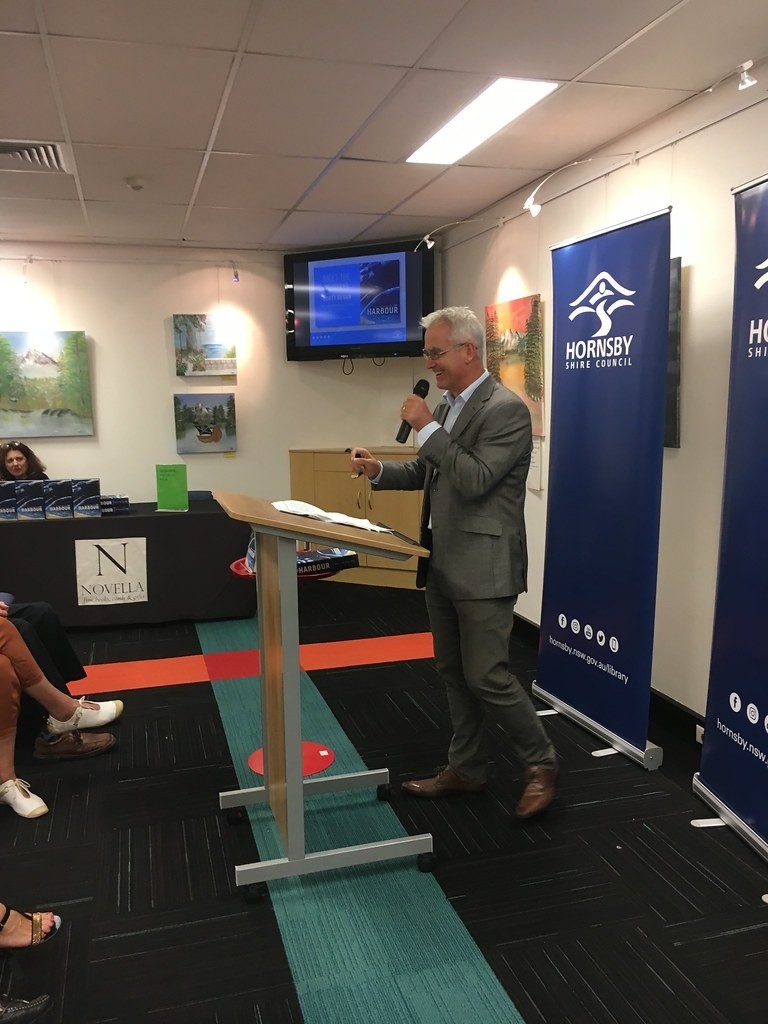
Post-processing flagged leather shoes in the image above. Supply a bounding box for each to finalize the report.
[515,763,561,818]
[400,765,487,797]
[32,726,116,758]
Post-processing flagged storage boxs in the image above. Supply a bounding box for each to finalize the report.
[296,547,358,575]
[246,530,257,574]
[0,478,130,520]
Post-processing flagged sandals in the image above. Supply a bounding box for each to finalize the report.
[0,901,62,947]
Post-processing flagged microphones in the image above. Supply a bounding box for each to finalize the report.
[396,379,430,444]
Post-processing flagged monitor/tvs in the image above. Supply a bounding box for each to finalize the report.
[284,237,436,361]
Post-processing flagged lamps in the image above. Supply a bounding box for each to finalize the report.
[523,150,637,217]
[230,259,239,282]
[710,55,768,91]
[414,214,506,252]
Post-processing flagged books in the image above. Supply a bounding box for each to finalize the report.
[245,529,257,573]
[297,548,360,574]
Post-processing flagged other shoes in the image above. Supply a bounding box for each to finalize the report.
[47,695,126,733]
[0,991,53,1024]
[0,774,49,819]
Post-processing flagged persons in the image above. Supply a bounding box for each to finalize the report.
[0,598,123,1024]
[350,307,562,820]
[0,441,49,481]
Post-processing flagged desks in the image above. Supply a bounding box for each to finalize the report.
[0,500,257,629]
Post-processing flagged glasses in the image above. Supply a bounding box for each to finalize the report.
[0,441,21,451]
[423,341,479,361]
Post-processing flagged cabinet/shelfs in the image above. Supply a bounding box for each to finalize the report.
[289,446,426,591]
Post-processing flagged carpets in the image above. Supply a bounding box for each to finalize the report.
[65,632,434,696]
[195,612,526,1024]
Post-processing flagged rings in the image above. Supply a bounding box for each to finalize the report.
[403,407,406,411]
